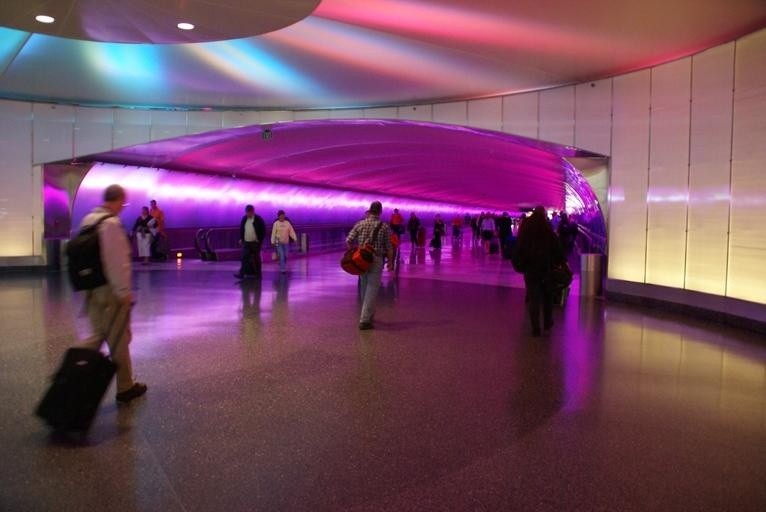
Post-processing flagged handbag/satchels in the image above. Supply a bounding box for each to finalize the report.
[341,243,376,275]
[66,224,107,292]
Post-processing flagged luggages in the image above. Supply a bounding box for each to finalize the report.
[34,299,136,441]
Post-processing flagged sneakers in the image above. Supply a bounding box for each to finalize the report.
[116,382,147,402]
[358,323,374,330]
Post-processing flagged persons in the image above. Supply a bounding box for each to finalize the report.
[346,200,393,331]
[273,210,295,243]
[232,204,266,279]
[511,206,561,338]
[129,206,158,265]
[342,208,579,284]
[75,184,148,402]
[149,200,164,260]
[270,211,298,275]
